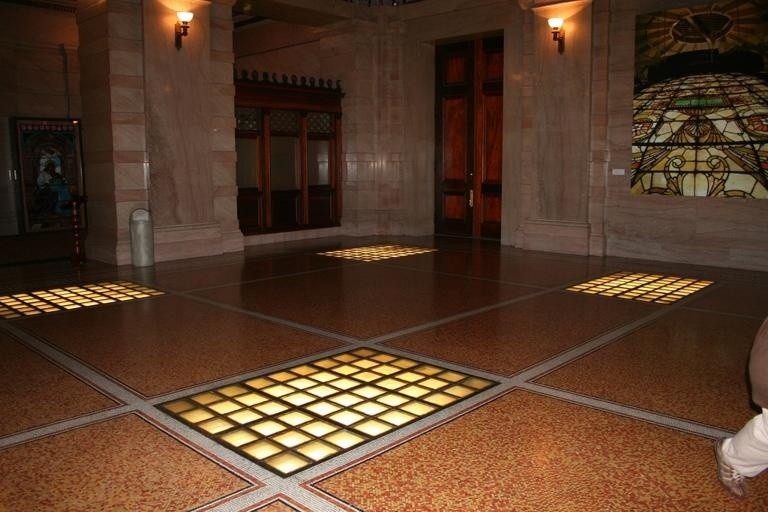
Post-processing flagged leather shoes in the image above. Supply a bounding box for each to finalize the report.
[714,437,746,498]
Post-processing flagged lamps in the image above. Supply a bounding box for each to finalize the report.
[548,17,565,53]
[175,10,194,50]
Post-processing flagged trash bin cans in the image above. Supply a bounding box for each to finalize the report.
[130,208,154,267]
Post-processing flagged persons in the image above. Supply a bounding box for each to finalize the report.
[31,158,68,229]
[712,317,768,499]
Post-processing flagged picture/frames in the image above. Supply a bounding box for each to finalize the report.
[9,113,88,232]
